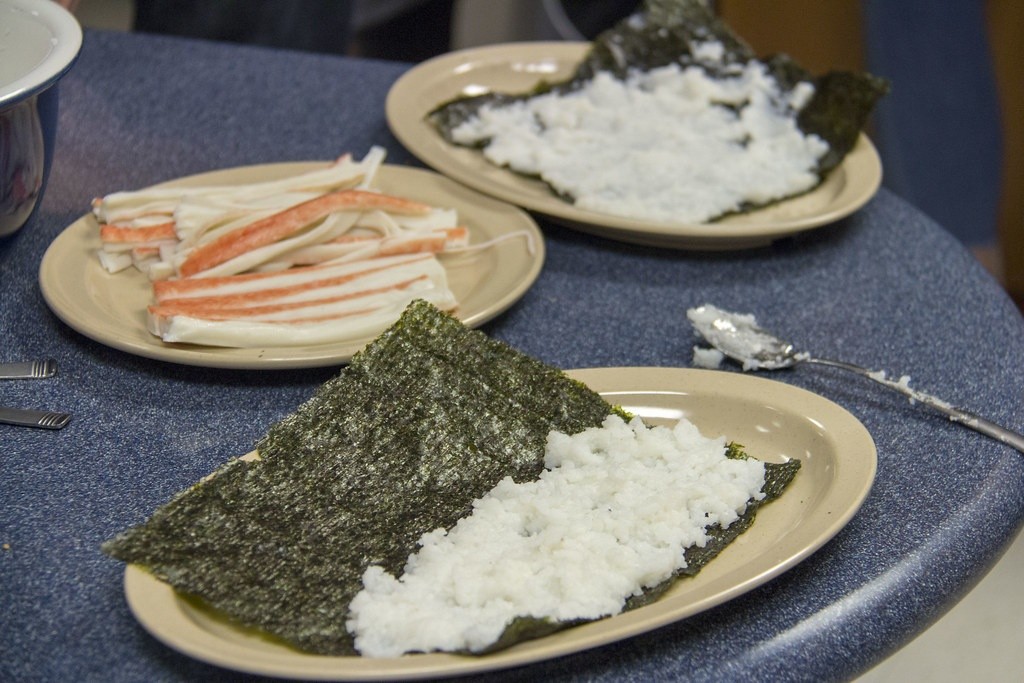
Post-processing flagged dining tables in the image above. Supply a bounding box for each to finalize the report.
[0,20,1023,683]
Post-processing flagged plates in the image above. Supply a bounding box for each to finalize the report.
[386,40,880,257]
[42,157,543,371]
[122,365,877,679]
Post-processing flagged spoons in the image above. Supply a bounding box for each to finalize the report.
[689,303,1022,464]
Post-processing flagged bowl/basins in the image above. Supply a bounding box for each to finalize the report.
[2,6,81,239]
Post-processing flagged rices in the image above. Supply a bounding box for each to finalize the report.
[345,413,767,657]
[450,40,831,224]
